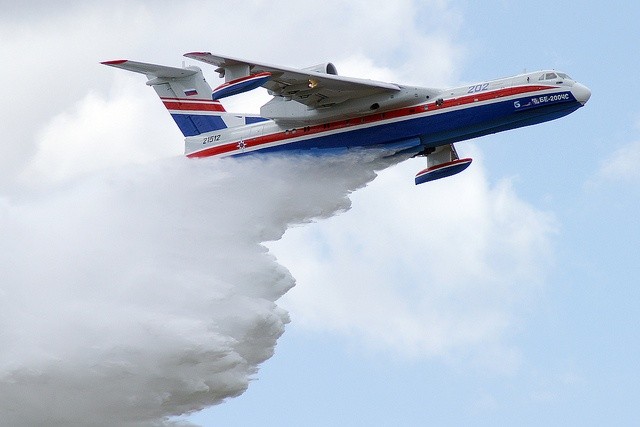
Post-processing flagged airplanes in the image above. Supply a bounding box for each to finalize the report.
[101,53,591,186]
